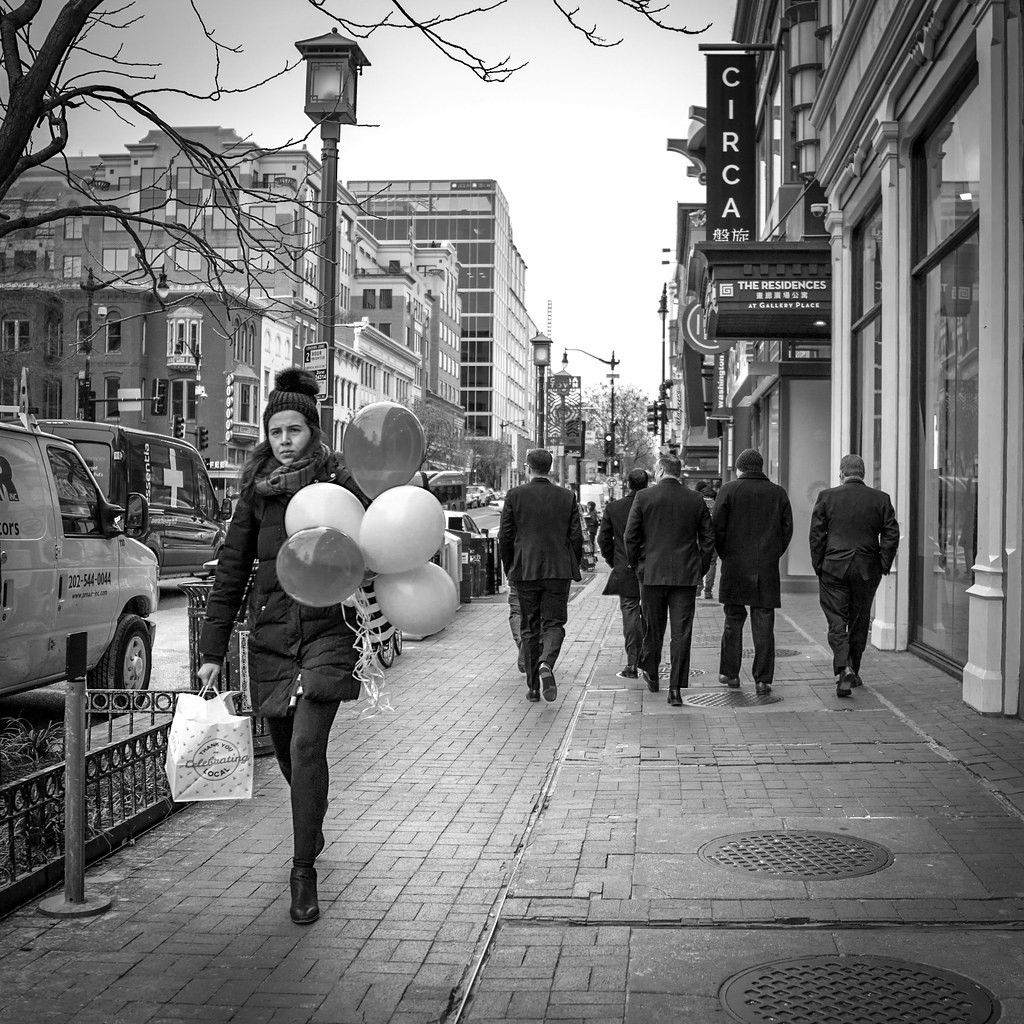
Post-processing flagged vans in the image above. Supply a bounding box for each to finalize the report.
[0,366,161,720]
[1,420,234,593]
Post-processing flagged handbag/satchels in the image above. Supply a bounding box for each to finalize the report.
[165,681,256,802]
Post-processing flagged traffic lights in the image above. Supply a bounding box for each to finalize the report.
[155,378,166,412]
[604,433,615,457]
[173,414,185,439]
[611,455,619,473]
[596,460,606,474]
[195,426,208,451]
[647,405,657,436]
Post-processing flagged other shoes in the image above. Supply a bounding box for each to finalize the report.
[517,643,526,672]
[539,663,558,703]
[526,688,539,704]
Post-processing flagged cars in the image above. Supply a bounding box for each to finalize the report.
[443,510,485,563]
[407,469,492,514]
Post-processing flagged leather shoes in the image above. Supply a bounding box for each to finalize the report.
[313,802,331,857]
[756,681,771,694]
[718,673,740,688]
[291,855,319,922]
[666,689,683,706]
[622,664,638,677]
[835,668,861,697]
[644,668,659,692]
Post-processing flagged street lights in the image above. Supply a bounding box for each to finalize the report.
[79,264,171,422]
[528,332,554,449]
[295,25,372,454]
[562,346,620,501]
[657,282,670,455]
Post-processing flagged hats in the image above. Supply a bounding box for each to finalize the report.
[734,449,763,474]
[263,368,323,428]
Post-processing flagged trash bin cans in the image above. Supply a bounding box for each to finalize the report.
[472,537,495,596]
[178,580,273,758]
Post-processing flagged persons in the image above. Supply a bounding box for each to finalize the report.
[586,501,599,543]
[809,455,900,697]
[713,449,794,695]
[624,455,713,705]
[695,481,717,599]
[501,449,584,702]
[951,477,974,550]
[597,468,649,680]
[198,369,375,925]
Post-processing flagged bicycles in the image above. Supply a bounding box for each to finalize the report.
[371,629,403,668]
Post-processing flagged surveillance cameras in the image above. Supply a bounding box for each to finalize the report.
[811,206,824,218]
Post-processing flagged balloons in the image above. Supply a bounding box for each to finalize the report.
[285,483,366,568]
[277,526,365,608]
[342,402,425,498]
[374,562,458,636]
[360,485,445,574]
